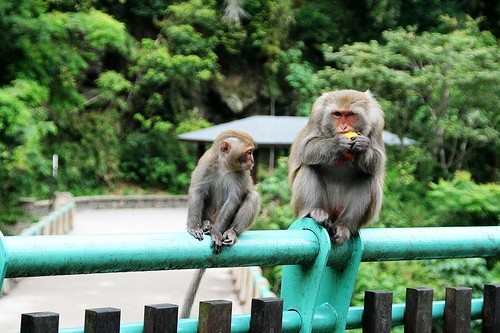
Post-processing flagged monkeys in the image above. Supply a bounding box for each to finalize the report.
[287,88,387,245]
[175,130,262,320]
